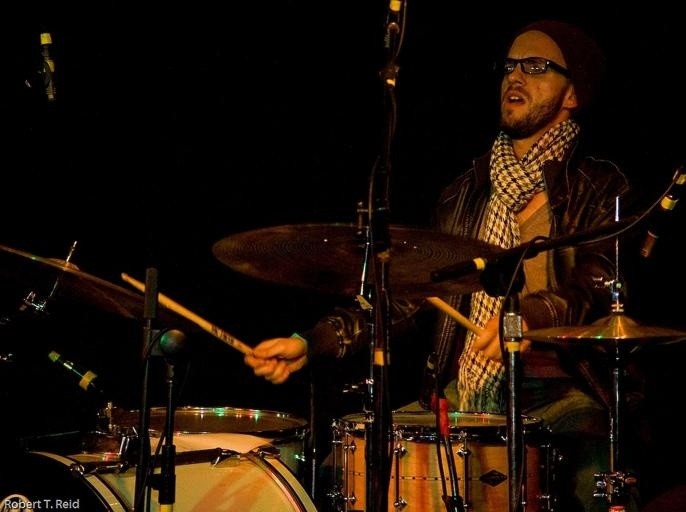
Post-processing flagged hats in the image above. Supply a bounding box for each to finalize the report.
[515,21,609,106]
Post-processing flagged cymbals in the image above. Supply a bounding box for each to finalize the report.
[212,223,508,300]
[0,244,173,320]
[520,317,685,349]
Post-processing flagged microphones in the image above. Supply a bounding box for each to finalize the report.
[158,365,175,504]
[40,32,57,101]
[378,0,402,77]
[640,169,685,258]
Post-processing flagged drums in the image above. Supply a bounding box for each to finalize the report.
[28,447,318,512]
[112,405,309,474]
[342,411,544,512]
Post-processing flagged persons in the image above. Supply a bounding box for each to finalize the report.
[244,20,635,512]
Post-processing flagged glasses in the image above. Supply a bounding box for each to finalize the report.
[498,57,569,76]
